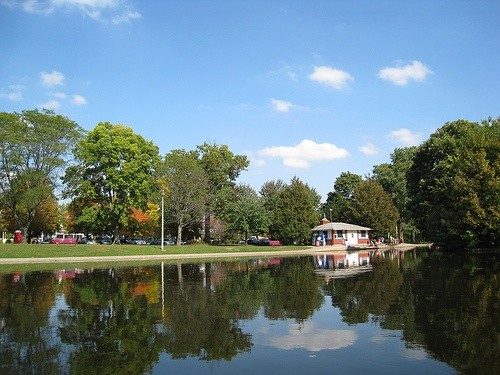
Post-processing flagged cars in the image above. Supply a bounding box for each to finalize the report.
[238,236,271,245]
[33,233,190,246]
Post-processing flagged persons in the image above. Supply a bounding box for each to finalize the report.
[368,250,405,261]
[367,235,403,247]
